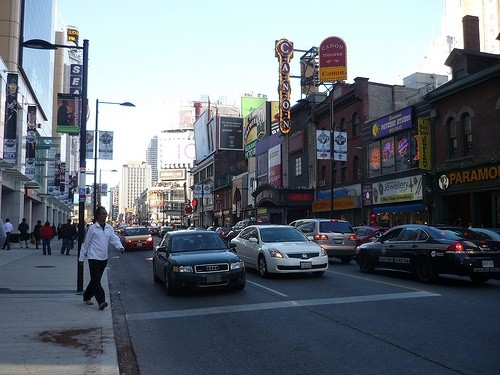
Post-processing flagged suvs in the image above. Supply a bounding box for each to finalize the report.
[288,218,357,265]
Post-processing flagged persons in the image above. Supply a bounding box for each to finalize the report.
[34,220,42,249]
[57,218,90,250]
[58,100,74,125]
[62,219,76,256]
[27,111,36,131]
[0,216,14,251]
[51,223,56,238]
[79,206,124,310]
[18,218,29,248]
[41,221,54,255]
[9,79,17,95]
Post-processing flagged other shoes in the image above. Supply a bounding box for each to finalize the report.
[99,302,108,310]
[83,297,93,305]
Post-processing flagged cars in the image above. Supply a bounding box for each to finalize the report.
[188,220,271,245]
[152,230,246,296]
[352,224,500,284]
[148,226,174,238]
[230,224,329,279]
[352,226,390,248]
[468,228,500,241]
[120,227,153,252]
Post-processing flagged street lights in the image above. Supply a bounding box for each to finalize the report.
[94,99,137,222]
[21,38,90,294]
[296,99,335,218]
[99,169,118,208]
